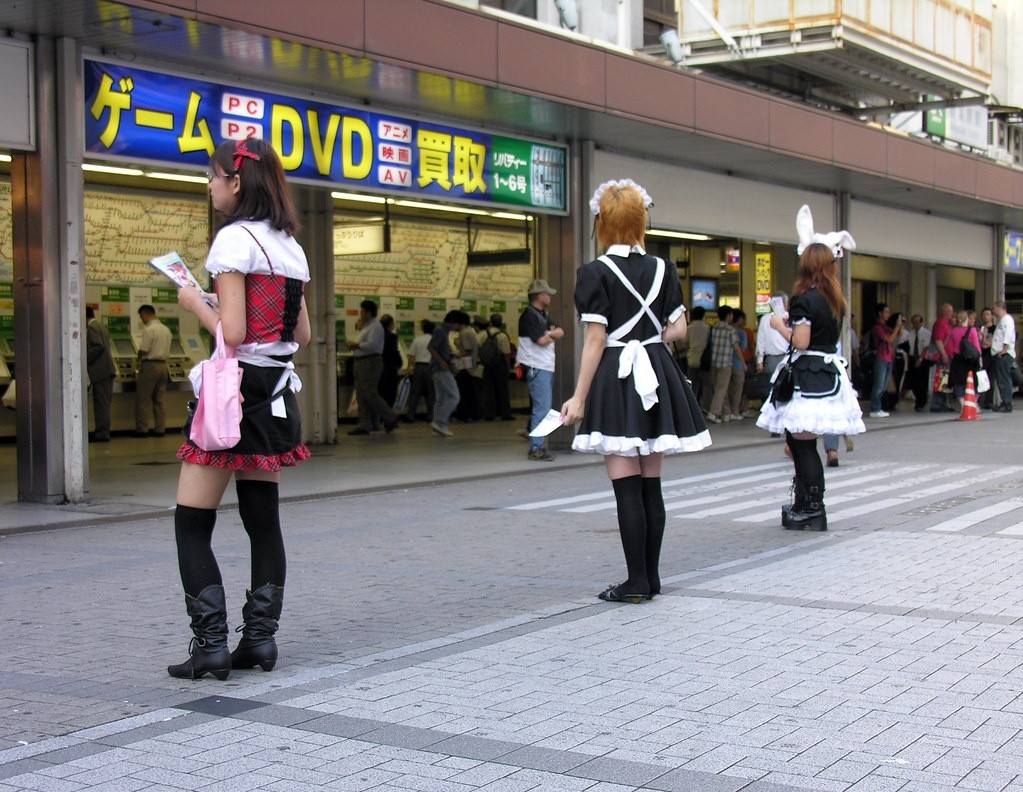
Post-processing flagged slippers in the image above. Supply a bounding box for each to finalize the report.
[598,582,656,603]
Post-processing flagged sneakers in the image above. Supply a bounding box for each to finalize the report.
[528,447,556,461]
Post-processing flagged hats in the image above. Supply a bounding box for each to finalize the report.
[527,279,557,295]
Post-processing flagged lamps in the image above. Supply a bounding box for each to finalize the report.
[555,0,579,31]
[660,29,686,69]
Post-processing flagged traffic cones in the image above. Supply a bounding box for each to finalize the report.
[957,370,982,421]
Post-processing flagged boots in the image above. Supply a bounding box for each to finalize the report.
[168,584,232,681]
[781,474,826,530]
[230,582,285,671]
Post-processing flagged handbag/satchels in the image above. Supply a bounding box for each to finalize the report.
[958,326,980,366]
[700,326,714,372]
[933,366,955,393]
[393,375,411,412]
[189,321,245,450]
[921,344,943,361]
[771,334,795,403]
[976,369,990,392]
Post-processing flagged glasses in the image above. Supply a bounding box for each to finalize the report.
[205,171,236,185]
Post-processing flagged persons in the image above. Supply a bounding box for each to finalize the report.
[756,204,866,530]
[133,304,173,438]
[346,300,516,437]
[167,137,311,680]
[516,278,564,463]
[86,305,117,443]
[681,305,792,426]
[851,301,1016,418]
[561,178,713,602]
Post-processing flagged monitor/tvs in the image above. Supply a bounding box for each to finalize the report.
[114,339,136,355]
[7,338,15,352]
[336,340,350,352]
[168,338,185,355]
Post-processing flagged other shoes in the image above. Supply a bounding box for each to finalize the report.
[826,450,839,467]
[429,422,454,437]
[131,430,165,438]
[347,415,416,435]
[693,398,758,422]
[785,445,794,460]
[866,385,1023,418]
[88,434,111,442]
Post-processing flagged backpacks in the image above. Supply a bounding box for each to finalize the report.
[479,330,504,366]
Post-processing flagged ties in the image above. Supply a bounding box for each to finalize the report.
[913,329,919,358]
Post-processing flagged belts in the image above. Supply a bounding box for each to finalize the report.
[142,358,166,364]
